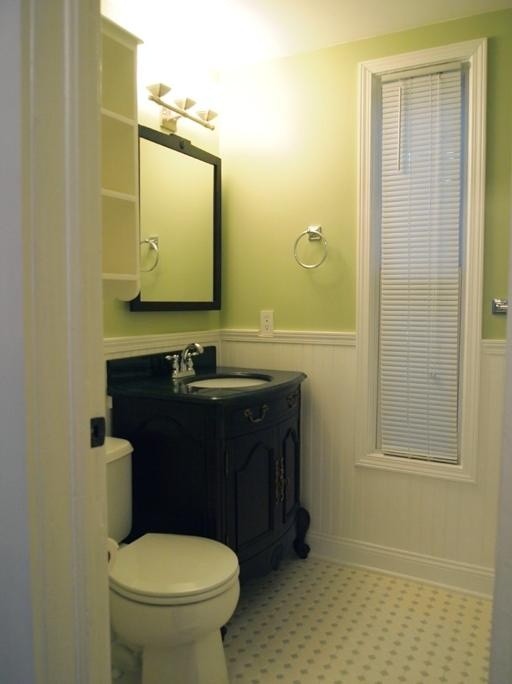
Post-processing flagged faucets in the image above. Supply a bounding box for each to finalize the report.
[180,342,205,374]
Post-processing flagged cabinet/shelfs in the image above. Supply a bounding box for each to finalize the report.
[110,412,300,566]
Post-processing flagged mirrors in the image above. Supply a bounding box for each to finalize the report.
[130,124,222,309]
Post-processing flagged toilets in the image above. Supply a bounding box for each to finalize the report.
[103,436,241,684]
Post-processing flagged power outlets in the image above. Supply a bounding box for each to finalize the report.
[262,311,273,336]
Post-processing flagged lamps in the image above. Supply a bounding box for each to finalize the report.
[147,69,217,133]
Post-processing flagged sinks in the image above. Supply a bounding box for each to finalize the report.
[183,373,274,388]
